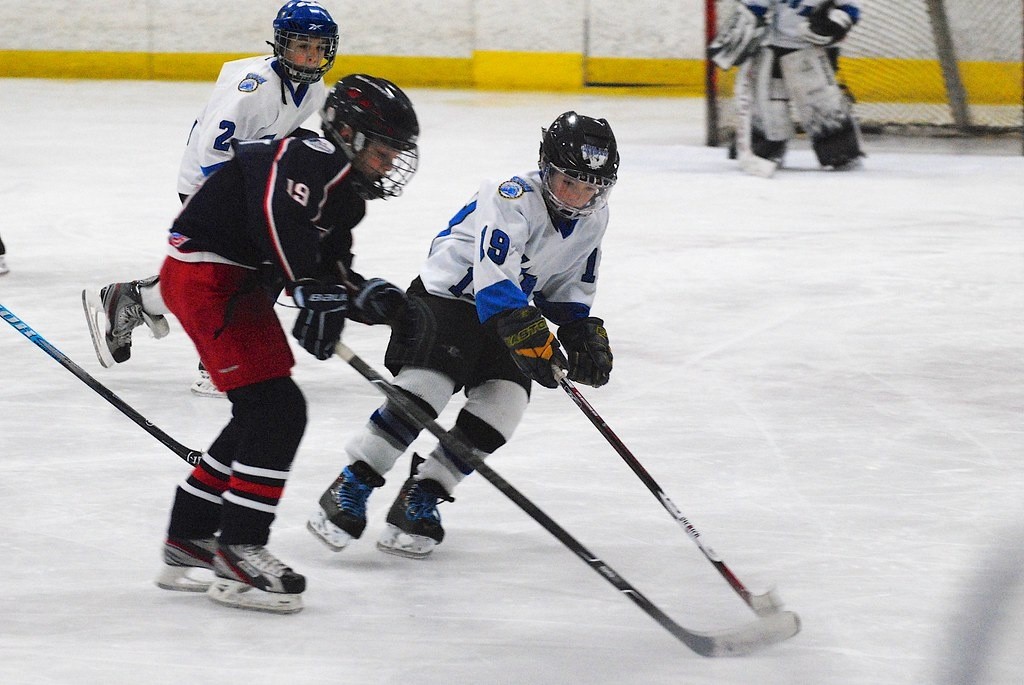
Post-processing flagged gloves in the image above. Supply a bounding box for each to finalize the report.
[496,306,570,389]
[557,317,613,388]
[293,283,348,361]
[354,277,413,343]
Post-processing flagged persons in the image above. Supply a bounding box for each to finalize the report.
[705,0,867,176]
[158,74,419,614]
[308,106,620,559]
[81,0,339,397]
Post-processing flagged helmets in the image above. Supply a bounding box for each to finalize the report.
[537,112,620,222]
[272,1,339,84]
[321,73,419,200]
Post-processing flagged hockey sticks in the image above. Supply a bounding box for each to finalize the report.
[0,303,207,464]
[552,364,828,617]
[335,343,802,659]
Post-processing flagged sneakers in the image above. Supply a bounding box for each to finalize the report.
[377,452,455,558]
[153,535,221,592]
[206,542,306,615]
[81,274,169,367]
[190,358,228,398]
[308,459,386,547]
